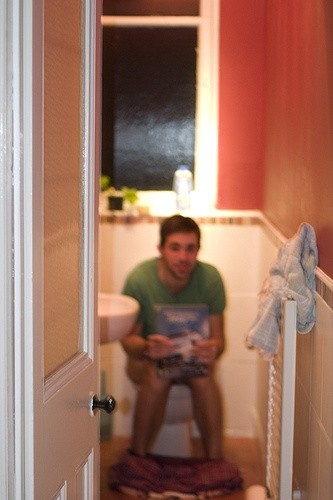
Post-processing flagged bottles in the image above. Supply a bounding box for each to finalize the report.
[171,164,193,211]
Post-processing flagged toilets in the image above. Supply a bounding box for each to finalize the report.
[147,382,195,458]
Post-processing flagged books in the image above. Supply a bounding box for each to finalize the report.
[151,302,210,381]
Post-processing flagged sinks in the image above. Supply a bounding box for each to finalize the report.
[99,294,137,315]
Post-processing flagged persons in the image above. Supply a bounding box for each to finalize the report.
[118,214,225,464]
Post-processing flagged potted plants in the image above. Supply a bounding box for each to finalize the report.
[100,175,138,210]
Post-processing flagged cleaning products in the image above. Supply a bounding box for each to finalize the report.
[172,159,193,210]
